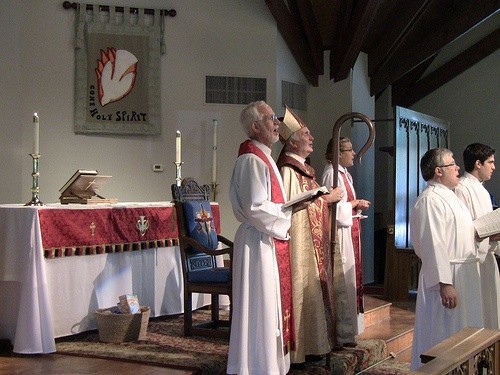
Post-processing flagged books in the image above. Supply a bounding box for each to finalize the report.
[283,186,330,208]
[59,168,99,194]
[474,208,500,238]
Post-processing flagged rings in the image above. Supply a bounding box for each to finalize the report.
[446,301,449,304]
[340,194,342,198]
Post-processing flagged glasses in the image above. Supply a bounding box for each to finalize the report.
[263,116,277,120]
[438,163,455,167]
[340,149,353,152]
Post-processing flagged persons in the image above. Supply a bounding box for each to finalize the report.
[410,148,485,371]
[321,137,371,352]
[276,104,344,371]
[227,101,311,375]
[452,142,500,330]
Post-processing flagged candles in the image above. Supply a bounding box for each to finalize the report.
[175,130,182,162]
[33,113,39,154]
[212,119,217,182]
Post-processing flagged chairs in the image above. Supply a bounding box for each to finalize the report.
[172,177,234,337]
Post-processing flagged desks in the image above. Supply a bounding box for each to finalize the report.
[0,200,230,355]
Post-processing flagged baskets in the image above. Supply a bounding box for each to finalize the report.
[95,306,151,342]
